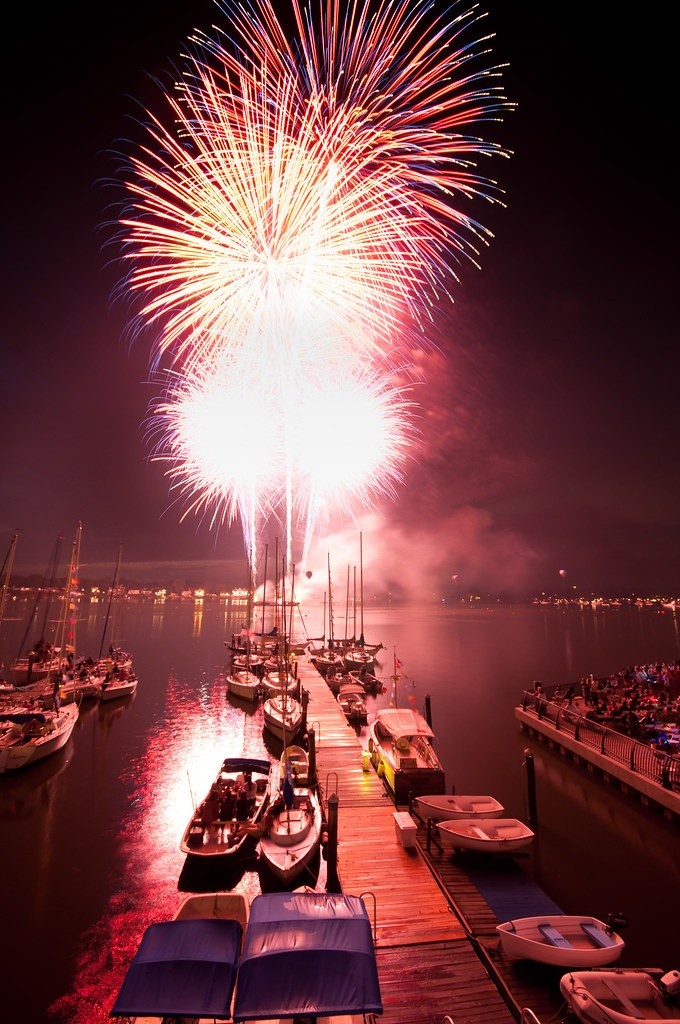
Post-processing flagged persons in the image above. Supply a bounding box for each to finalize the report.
[359,665,366,681]
[231,633,247,648]
[78,651,135,680]
[319,646,326,658]
[196,776,257,832]
[531,660,680,751]
[329,664,336,681]
[23,696,35,708]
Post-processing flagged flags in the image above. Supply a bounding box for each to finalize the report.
[60,565,79,684]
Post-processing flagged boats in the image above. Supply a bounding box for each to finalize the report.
[558,967,680,1024]
[415,793,507,825]
[176,756,274,862]
[227,886,387,1024]
[109,892,250,1023]
[493,913,627,971]
[431,817,534,851]
[366,707,446,799]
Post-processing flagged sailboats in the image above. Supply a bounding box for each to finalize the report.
[0,520,139,772]
[224,534,389,886]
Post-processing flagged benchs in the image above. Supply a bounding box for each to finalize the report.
[539,924,573,948]
[449,800,463,811]
[580,924,613,949]
[603,978,645,1018]
[195,818,252,825]
[472,825,490,840]
[342,702,361,705]
